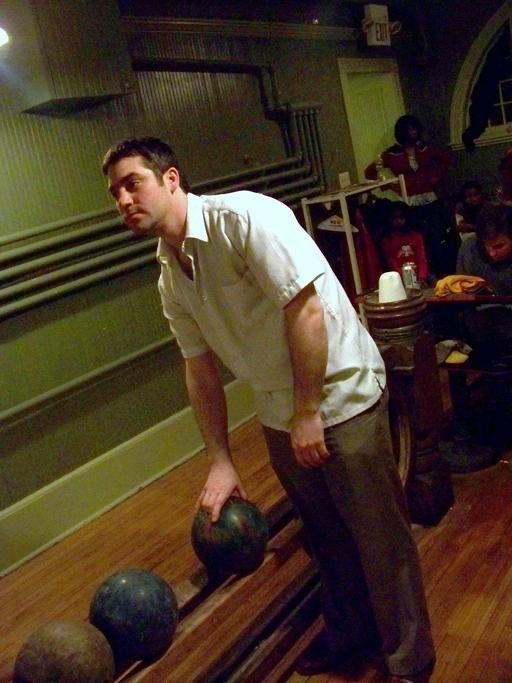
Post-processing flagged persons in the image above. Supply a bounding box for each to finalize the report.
[363,116,512,371]
[102,136,436,683]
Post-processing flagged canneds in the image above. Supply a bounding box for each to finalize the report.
[402,261,417,288]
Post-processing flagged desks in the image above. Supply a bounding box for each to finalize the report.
[418,279,511,475]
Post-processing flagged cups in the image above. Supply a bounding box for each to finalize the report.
[379,272,407,302]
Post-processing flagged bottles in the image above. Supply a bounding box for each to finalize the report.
[376,155,385,181]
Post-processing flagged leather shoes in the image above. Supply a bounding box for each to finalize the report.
[386,655,436,683]
[295,644,341,676]
